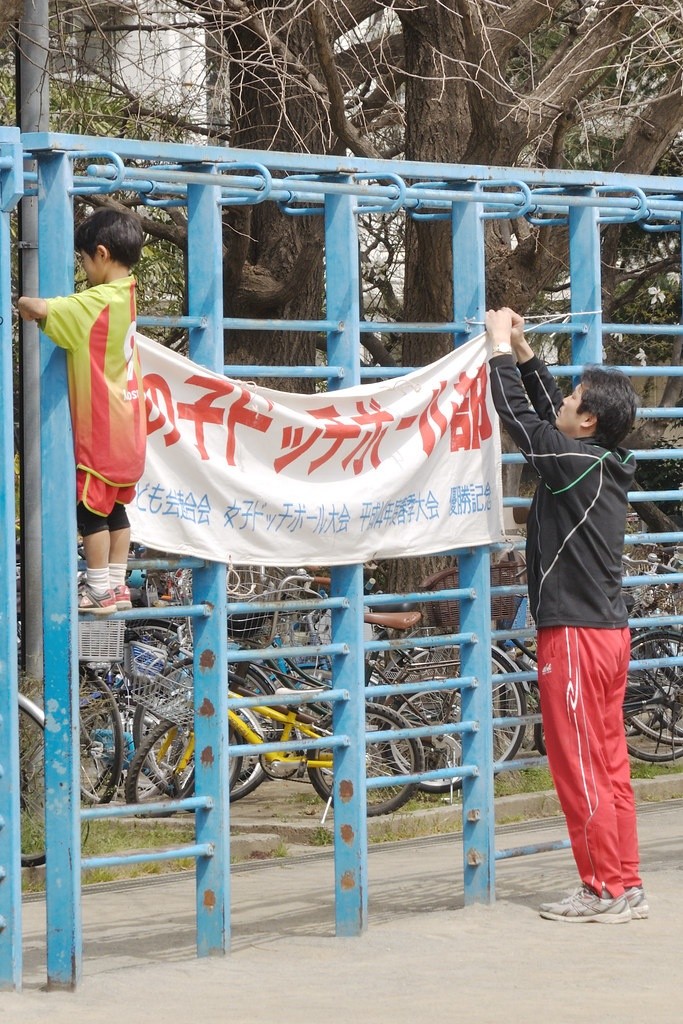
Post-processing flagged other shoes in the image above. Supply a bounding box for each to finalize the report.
[78,580,116,614]
[114,584,132,609]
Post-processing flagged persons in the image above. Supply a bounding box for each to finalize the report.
[15,206,149,615]
[480,306,651,926]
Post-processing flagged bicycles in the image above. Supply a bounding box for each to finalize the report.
[15,537,683,828]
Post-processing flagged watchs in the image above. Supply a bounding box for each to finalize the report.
[491,342,513,354]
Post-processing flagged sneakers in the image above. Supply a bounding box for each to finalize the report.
[626,886,649,919]
[538,890,631,924]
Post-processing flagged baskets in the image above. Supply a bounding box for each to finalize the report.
[68,554,654,725]
